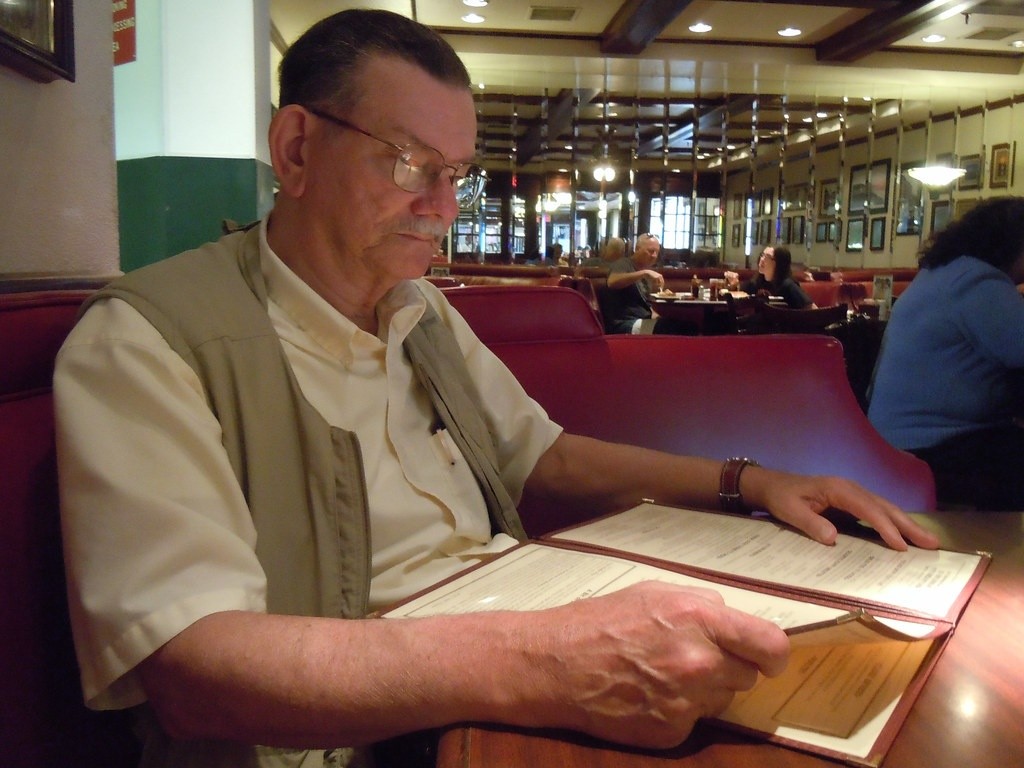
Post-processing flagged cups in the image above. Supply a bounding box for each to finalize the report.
[691,279,703,297]
[710,279,727,298]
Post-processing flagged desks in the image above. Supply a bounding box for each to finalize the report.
[437,505,1024,768]
[645,289,779,334]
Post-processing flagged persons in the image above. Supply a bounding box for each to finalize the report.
[52,8,938,768]
[545,237,634,267]
[581,238,625,268]
[868,196,1024,512]
[607,233,665,320]
[724,242,818,310]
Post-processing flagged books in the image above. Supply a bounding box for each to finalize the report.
[368,496,992,768]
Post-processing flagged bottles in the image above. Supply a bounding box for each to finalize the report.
[877,299,889,316]
[698,286,705,298]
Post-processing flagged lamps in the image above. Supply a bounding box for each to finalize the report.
[906,161,966,193]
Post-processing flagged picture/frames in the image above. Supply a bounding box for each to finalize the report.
[895,159,923,235]
[989,140,1015,187]
[869,216,884,249]
[929,201,953,236]
[0,0,80,86]
[935,149,955,189]
[698,202,719,244]
[729,179,840,247]
[959,152,985,191]
[845,217,864,252]
[849,159,890,214]
[956,198,984,218]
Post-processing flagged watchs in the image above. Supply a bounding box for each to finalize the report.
[719,456,761,515]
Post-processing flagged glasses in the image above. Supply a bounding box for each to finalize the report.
[759,251,775,261]
[646,232,660,239]
[302,103,492,210]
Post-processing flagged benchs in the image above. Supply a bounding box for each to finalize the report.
[0,265,1024,768]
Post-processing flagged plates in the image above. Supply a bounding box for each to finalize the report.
[650,292,680,298]
